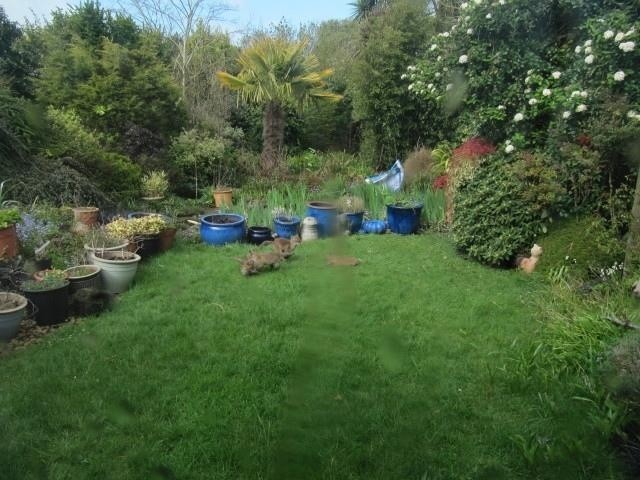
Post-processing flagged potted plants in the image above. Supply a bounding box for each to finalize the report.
[196,186,425,247]
[1,204,177,346]
